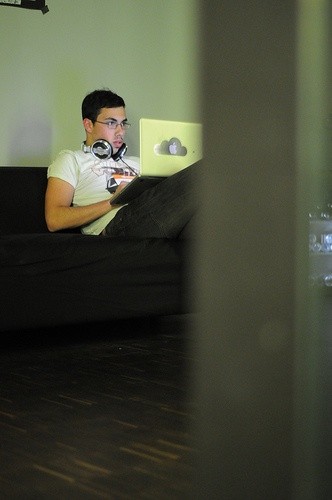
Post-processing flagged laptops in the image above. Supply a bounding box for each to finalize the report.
[109,119,202,205]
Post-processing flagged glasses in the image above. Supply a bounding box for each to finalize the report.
[94,121,130,129]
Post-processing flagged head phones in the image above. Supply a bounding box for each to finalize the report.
[80,141,128,161]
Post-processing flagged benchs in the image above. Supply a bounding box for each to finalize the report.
[0,167,194,328]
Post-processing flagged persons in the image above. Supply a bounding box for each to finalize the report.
[44,87,214,240]
